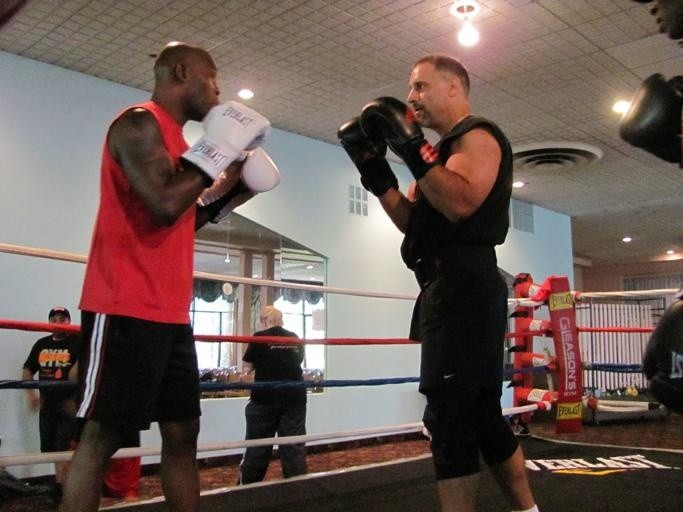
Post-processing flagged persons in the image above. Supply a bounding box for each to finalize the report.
[54,41,282,510]
[20,305,82,501]
[333,51,547,511]
[237,304,309,486]
[617,1,683,418]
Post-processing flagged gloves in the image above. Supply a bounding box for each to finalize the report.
[178,100,271,189]
[336,116,398,198]
[196,141,280,224]
[619,71,683,165]
[641,288,683,417]
[359,97,440,181]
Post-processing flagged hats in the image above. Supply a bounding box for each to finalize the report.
[48,306,69,318]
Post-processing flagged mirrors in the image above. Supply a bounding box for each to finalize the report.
[189,210,330,402]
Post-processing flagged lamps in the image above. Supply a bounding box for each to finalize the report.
[449,0,480,47]
